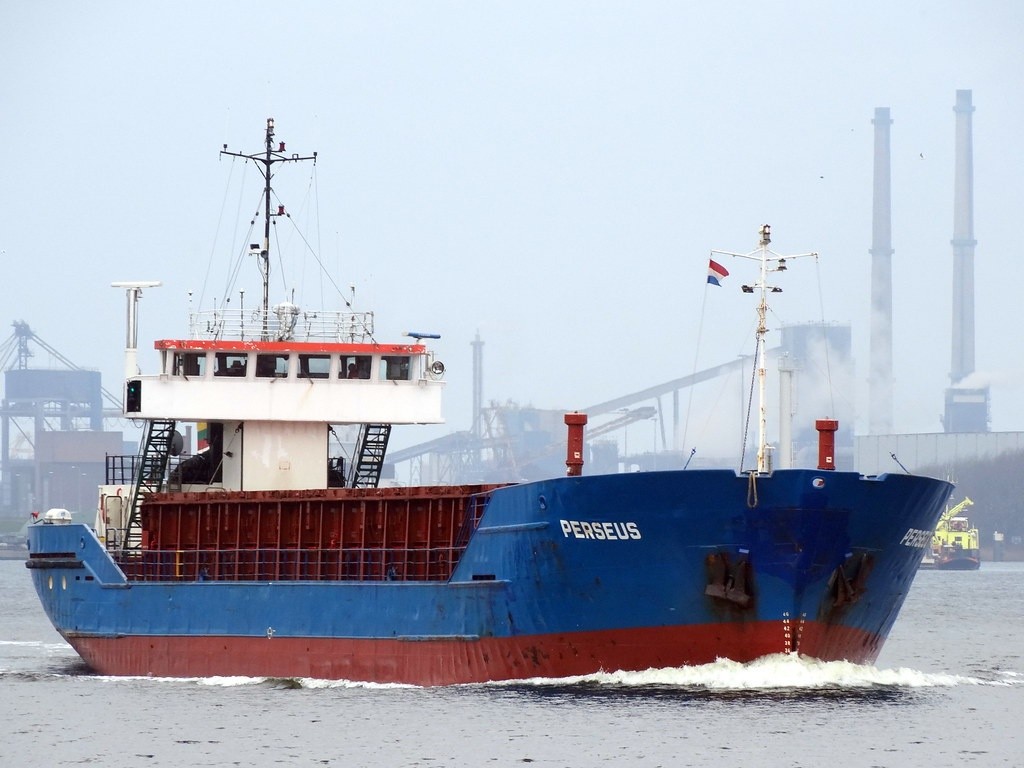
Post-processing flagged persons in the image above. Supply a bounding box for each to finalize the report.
[347,363,359,379]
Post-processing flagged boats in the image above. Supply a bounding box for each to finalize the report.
[927,471,981,570]
[21,115,957,687]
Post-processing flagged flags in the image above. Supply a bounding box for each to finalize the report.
[197,422,211,450]
[707,259,730,287]
